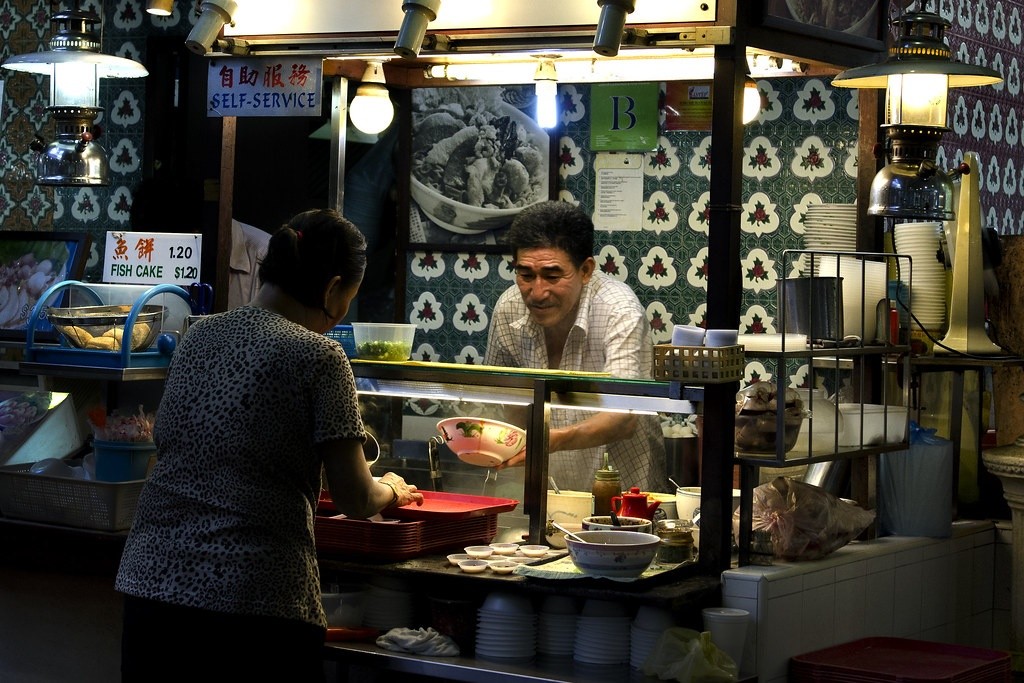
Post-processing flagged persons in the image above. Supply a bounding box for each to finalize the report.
[484,200,668,493]
[113,209,423,683]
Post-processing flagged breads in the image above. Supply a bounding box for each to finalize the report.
[62,322,152,351]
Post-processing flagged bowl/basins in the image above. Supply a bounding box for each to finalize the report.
[475,594,675,668]
[435,416,527,467]
[735,403,908,453]
[410,101,552,234]
[352,322,417,362]
[564,485,742,577]
[819,256,886,345]
[30,453,94,492]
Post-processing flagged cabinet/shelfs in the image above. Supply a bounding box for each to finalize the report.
[346,364,714,607]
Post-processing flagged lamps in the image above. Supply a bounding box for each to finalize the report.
[145,0,175,16]
[393,0,452,59]
[743,76,761,125]
[2,0,149,185]
[356,378,697,417]
[831,0,1004,220]
[593,0,654,57]
[186,0,249,56]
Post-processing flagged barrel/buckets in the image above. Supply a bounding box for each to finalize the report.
[90,439,157,482]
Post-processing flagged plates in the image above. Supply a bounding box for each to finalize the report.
[361,587,413,630]
[0,396,49,427]
[447,543,549,574]
[800,204,948,331]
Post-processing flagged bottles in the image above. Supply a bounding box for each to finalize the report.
[790,389,846,450]
[595,450,621,518]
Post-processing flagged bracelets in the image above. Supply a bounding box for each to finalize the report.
[380,482,399,506]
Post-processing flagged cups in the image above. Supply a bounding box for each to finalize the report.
[665,437,697,493]
[545,490,592,548]
[776,277,843,342]
[665,324,739,374]
[703,607,750,680]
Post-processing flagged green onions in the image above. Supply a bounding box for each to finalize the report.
[354,340,411,361]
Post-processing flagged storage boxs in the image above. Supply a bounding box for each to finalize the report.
[833,402,909,447]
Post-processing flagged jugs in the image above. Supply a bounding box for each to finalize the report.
[611,487,663,522]
